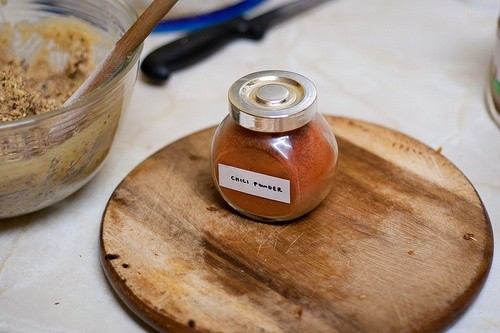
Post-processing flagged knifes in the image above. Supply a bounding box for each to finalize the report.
[138,0,340,86]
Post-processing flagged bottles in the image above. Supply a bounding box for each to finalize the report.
[209,68,339,224]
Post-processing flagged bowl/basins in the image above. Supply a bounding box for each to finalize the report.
[0,0,144,218]
